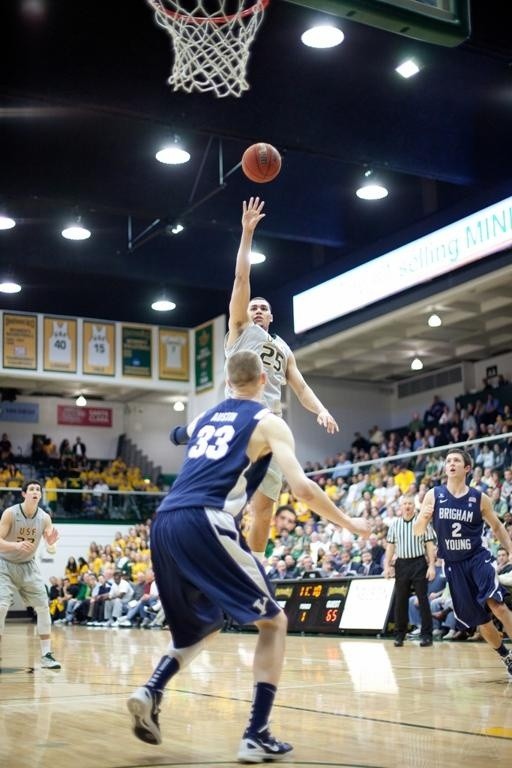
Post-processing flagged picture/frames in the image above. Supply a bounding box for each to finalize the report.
[158,327,190,382]
[83,319,116,377]
[194,320,213,394]
[122,322,152,376]
[43,316,78,374]
[2,312,38,369]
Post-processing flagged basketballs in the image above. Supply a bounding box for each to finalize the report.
[243,144,281,183]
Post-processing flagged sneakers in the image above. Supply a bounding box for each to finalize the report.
[125,685,162,745]
[499,649,511,682]
[41,652,61,670]
[53,617,171,629]
[237,728,294,764]
[394,638,404,647]
[407,627,483,641]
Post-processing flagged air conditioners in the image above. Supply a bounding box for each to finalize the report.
[390,361,476,429]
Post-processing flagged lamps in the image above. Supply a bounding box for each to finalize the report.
[410,349,424,371]
[0,200,16,230]
[301,12,345,49]
[427,305,442,327]
[61,207,92,241]
[155,132,191,165]
[0,262,22,294]
[75,393,87,407]
[249,233,266,265]
[151,288,176,312]
[356,161,388,200]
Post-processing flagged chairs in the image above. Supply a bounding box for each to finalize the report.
[0,454,167,520]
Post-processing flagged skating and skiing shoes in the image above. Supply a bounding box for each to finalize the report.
[419,637,433,646]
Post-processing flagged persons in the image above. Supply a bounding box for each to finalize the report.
[39,456,160,520]
[384,497,436,647]
[46,515,170,631]
[0,478,60,669]
[241,375,512,583]
[413,448,512,674]
[407,547,512,640]
[60,436,86,464]
[30,433,56,461]
[1,433,25,489]
[224,196,339,565]
[125,350,371,763]
